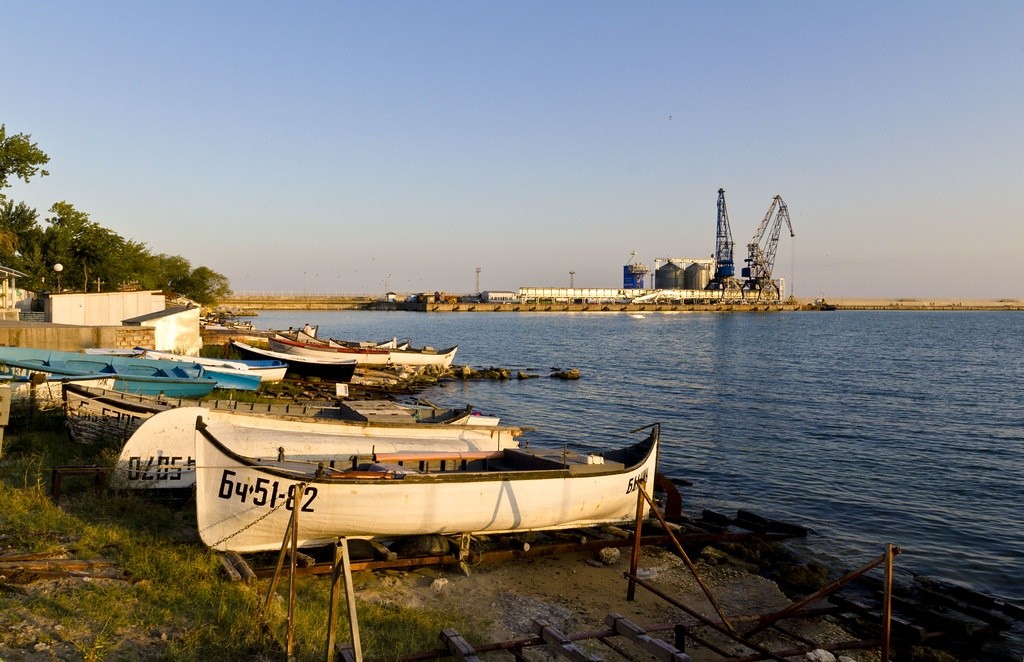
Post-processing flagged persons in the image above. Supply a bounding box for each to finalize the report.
[304,323,311,335]
[289,327,293,333]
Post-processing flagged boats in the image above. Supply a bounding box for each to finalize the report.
[61,376,502,449]
[228,337,360,384]
[274,331,459,367]
[267,335,391,370]
[276,325,320,339]
[108,405,538,494]
[192,415,662,554]
[1,369,119,412]
[0,346,263,391]
[0,352,219,400]
[79,344,289,386]
[199,312,257,332]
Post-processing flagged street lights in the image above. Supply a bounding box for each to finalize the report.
[475,267,482,296]
[569,271,576,289]
[54,263,64,294]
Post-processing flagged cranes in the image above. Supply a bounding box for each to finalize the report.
[703,186,740,305]
[737,194,796,302]
